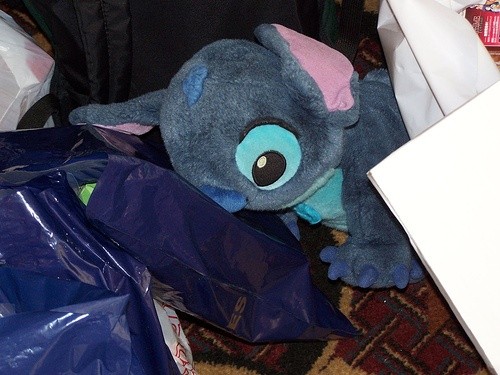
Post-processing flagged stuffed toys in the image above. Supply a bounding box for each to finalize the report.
[68,22,428,290]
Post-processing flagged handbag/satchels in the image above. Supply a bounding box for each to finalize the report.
[0,162,203,375]
[2,115,369,346]
[366,0,499,375]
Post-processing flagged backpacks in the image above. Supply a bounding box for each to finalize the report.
[20,2,361,155]
